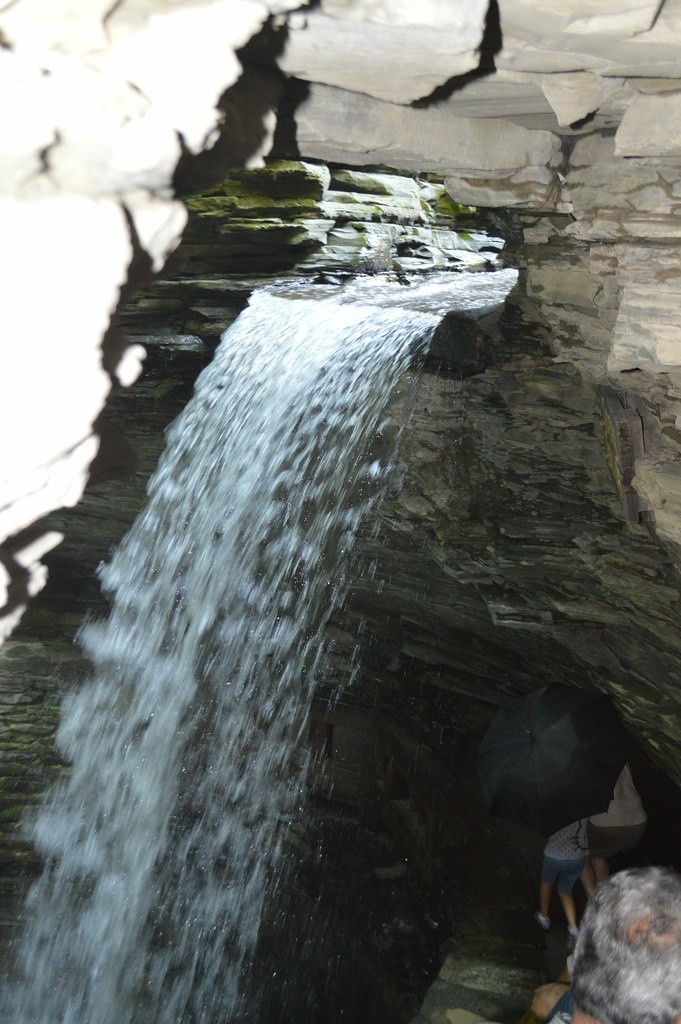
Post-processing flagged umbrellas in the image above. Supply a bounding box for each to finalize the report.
[468,682,632,838]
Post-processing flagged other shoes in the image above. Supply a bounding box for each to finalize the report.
[534,911,550,929]
[567,926,579,935]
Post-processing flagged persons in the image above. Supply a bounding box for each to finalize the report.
[536,760,648,955]
